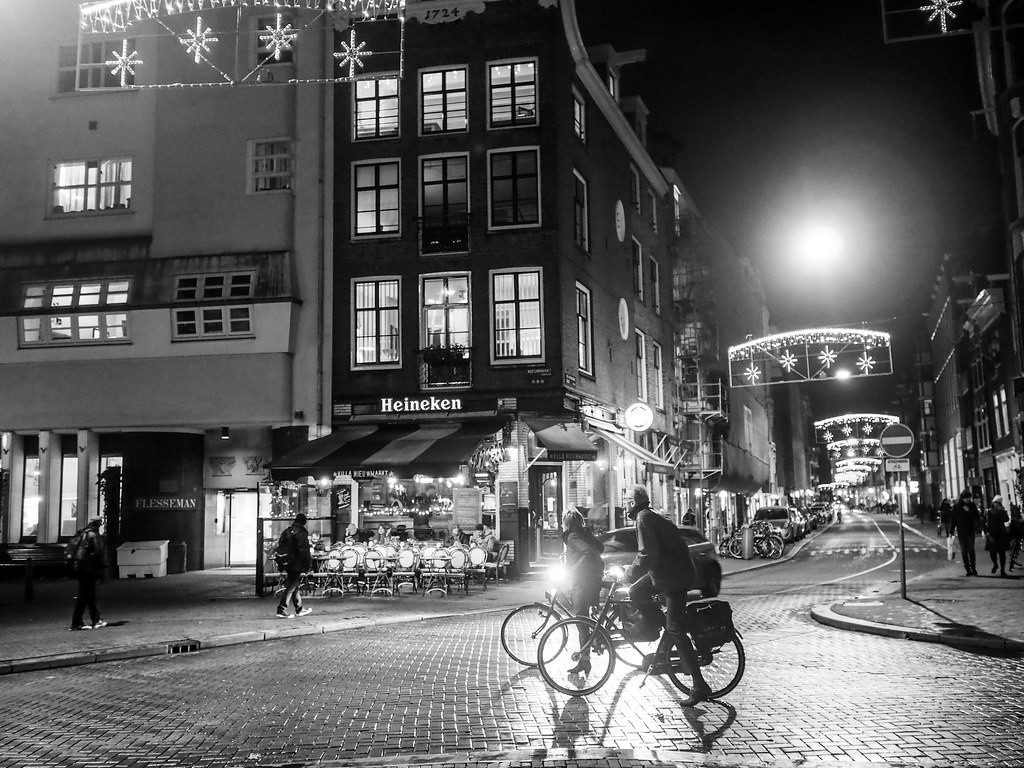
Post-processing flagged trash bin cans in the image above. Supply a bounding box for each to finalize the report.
[742,528,754,560]
[166,541,188,573]
[116,540,170,578]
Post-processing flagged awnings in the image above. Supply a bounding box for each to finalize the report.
[589,426,675,475]
[522,416,600,462]
[261,415,515,481]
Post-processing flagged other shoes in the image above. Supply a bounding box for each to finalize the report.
[276,612,294,618]
[1001,569,1008,579]
[966,572,971,577]
[92,621,107,629]
[295,609,312,617]
[70,623,91,630]
[991,564,1000,573]
[971,569,977,574]
[630,617,663,633]
[677,685,712,707]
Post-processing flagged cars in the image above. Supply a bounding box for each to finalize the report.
[557,526,722,609]
[754,506,794,544]
[790,501,834,542]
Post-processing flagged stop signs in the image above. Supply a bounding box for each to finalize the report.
[879,424,915,457]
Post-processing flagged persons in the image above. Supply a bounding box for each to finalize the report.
[368,524,392,545]
[469,523,496,552]
[616,483,712,708]
[69,516,108,630]
[980,494,1013,577]
[275,513,313,619]
[310,530,325,551]
[949,489,980,577]
[936,497,959,538]
[443,523,469,547]
[682,509,697,527]
[561,507,604,675]
[343,523,361,543]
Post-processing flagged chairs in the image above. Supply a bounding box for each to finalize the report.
[263,537,511,601]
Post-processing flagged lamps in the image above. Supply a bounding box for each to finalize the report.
[220,426,231,440]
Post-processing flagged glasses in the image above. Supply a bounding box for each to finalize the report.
[623,497,634,501]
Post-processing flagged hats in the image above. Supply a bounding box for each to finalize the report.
[295,514,307,524]
[991,495,1004,504]
[89,516,102,525]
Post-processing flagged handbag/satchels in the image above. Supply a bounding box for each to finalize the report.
[686,599,736,650]
[947,536,957,559]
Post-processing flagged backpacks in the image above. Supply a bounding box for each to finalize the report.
[275,530,302,571]
[63,528,95,578]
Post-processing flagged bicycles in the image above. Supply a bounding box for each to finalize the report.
[719,521,786,560]
[538,577,746,701]
[500,580,666,669]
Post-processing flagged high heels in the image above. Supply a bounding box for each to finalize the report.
[568,661,591,674]
